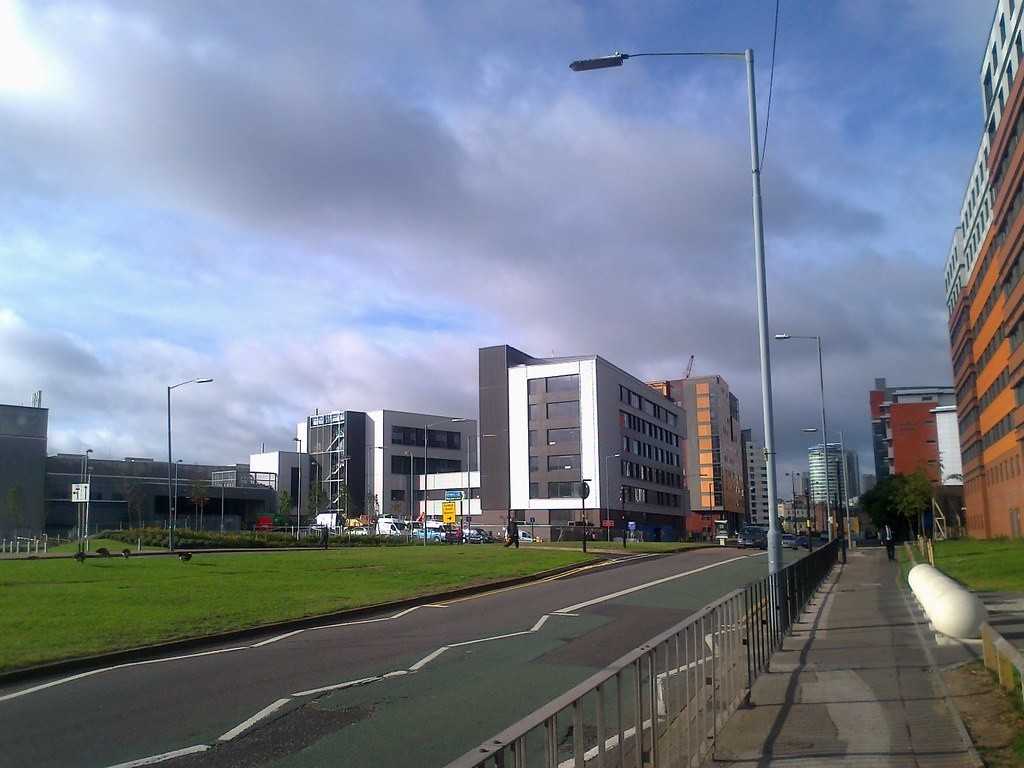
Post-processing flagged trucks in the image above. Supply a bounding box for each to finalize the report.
[504,529,536,543]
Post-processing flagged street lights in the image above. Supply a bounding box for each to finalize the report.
[785,471,800,537]
[423,418,467,545]
[311,462,318,524]
[291,437,301,541]
[773,333,832,542]
[467,433,497,544]
[605,453,621,540]
[710,482,722,540]
[404,451,414,537]
[173,459,183,530]
[802,428,853,551]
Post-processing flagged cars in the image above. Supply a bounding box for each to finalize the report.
[462,528,495,545]
[781,533,799,551]
[300,512,463,545]
[796,535,810,549]
[820,530,829,541]
[735,525,768,551]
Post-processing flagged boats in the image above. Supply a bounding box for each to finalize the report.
[77,449,95,553]
[167,378,213,553]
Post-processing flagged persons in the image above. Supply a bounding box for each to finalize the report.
[455,527,464,545]
[504,516,520,549]
[703,530,707,540]
[688,531,692,542]
[710,531,713,542]
[881,522,896,561]
